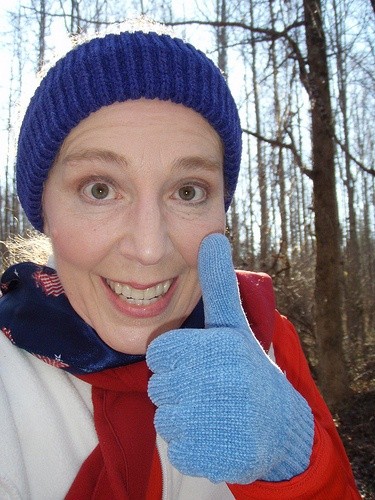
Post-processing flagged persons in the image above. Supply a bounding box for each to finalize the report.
[0,31,362,500]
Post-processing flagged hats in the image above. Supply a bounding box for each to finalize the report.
[16,16,243,232]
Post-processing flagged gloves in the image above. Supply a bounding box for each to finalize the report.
[144,232,316,487]
